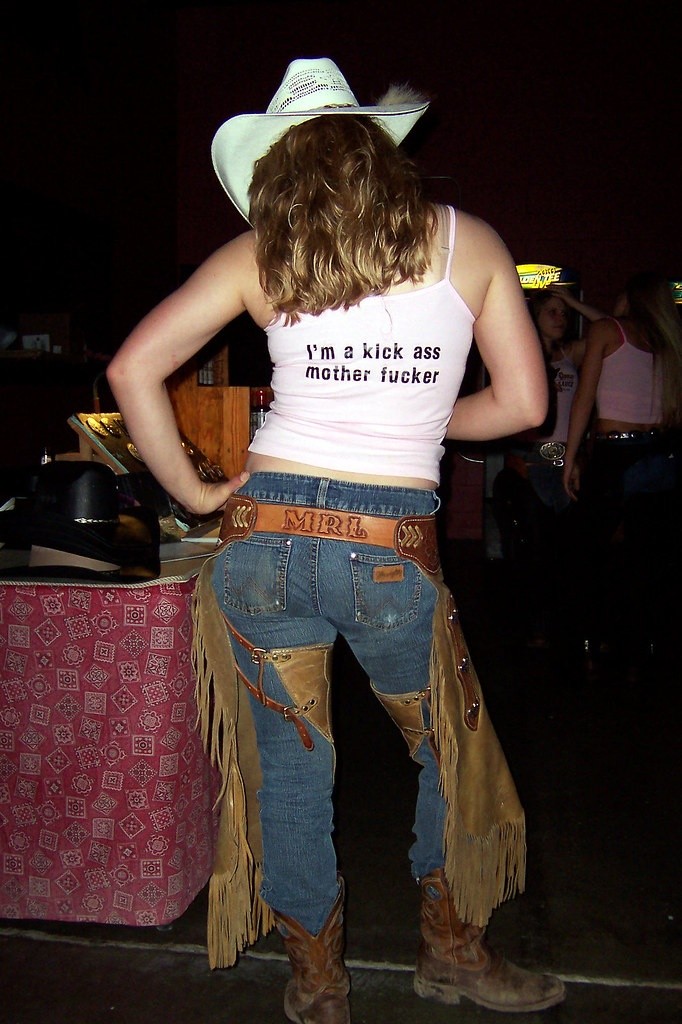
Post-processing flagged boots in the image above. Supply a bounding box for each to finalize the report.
[411,865,566,1013]
[257,871,352,1024]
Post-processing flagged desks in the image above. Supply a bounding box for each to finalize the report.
[0,539,223,931]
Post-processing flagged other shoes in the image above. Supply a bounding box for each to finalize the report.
[632,640,672,679]
[523,611,554,650]
[577,639,610,681]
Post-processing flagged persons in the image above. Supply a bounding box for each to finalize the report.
[487,284,606,651]
[561,270,682,684]
[105,55,566,1024]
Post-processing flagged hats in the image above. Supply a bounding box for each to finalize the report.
[0,455,163,584]
[211,58,430,230]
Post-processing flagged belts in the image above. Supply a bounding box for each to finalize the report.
[505,440,570,462]
[592,427,669,443]
